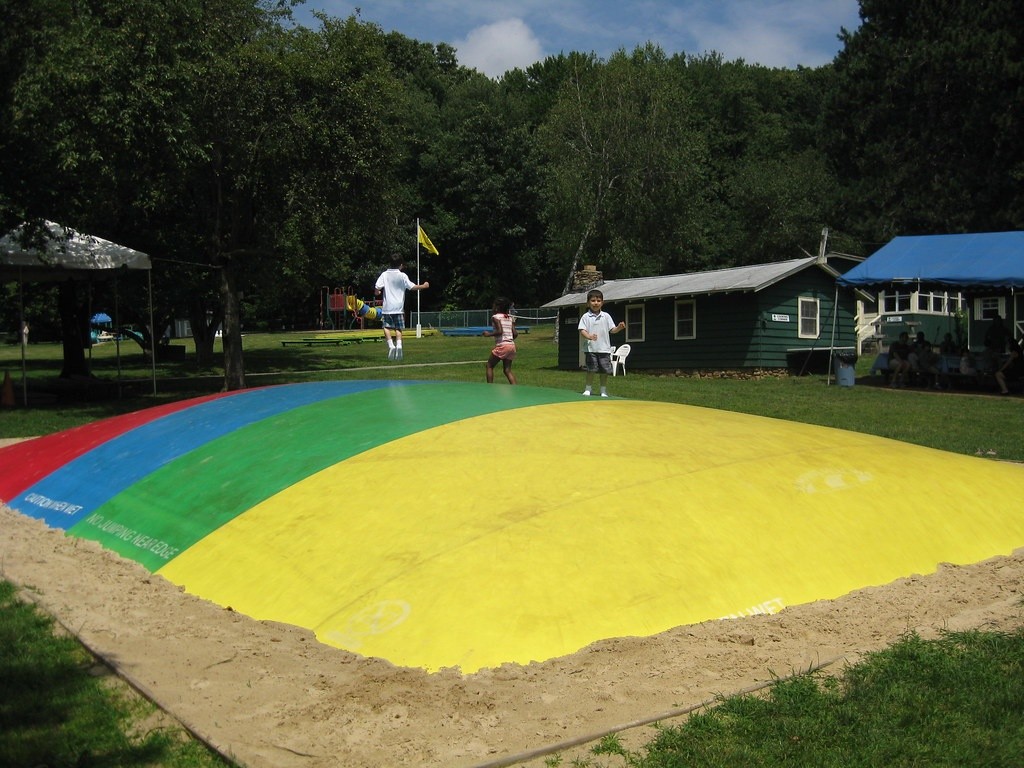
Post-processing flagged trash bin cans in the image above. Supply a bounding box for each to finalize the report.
[833,352,858,386]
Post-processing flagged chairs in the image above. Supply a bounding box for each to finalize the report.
[610,344,631,376]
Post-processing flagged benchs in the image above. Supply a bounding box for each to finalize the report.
[872,350,1024,393]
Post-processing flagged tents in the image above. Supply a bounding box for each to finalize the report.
[0,217,156,405]
[828,230,1024,387]
[91,312,112,335]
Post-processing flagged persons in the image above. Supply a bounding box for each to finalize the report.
[482,298,518,386]
[375,253,430,362]
[578,290,626,397]
[888,315,1024,394]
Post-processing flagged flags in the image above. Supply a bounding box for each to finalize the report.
[419,226,439,255]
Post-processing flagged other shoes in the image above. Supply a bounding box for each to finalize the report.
[600,390,608,397]
[583,389,592,396]
[1000,392,1009,395]
[395,345,403,360]
[388,346,396,362]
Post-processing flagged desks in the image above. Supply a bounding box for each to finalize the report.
[786,346,856,377]
